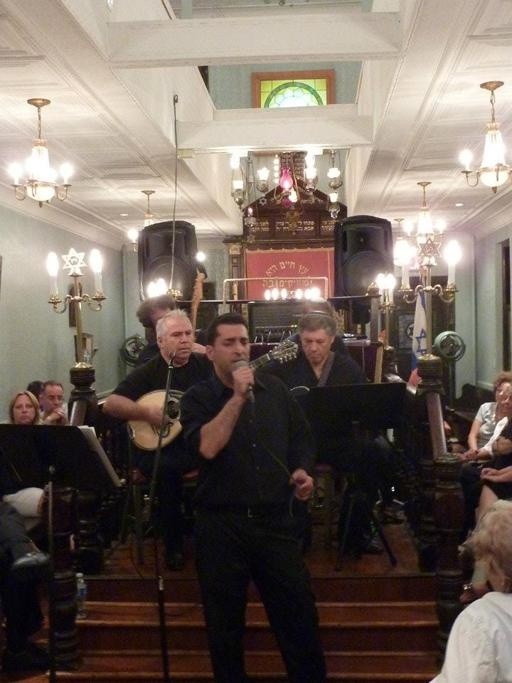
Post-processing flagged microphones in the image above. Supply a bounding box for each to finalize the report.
[232,361,262,411]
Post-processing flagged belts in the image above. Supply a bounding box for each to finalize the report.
[217,503,265,519]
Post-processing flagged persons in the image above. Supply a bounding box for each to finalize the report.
[0,295,509,683]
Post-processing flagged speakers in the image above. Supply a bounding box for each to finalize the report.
[137,219,200,312]
[332,215,395,315]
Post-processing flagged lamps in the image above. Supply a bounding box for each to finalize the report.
[375,272,398,350]
[231,148,343,228]
[128,228,139,253]
[392,240,463,361]
[141,190,156,226]
[46,246,107,369]
[401,181,449,249]
[458,81,512,194]
[7,99,76,208]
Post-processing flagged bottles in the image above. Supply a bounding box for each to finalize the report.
[77,573,86,619]
[144,495,150,521]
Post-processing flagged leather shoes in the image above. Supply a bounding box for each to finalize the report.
[3,642,49,671]
[345,538,384,556]
[165,543,185,569]
